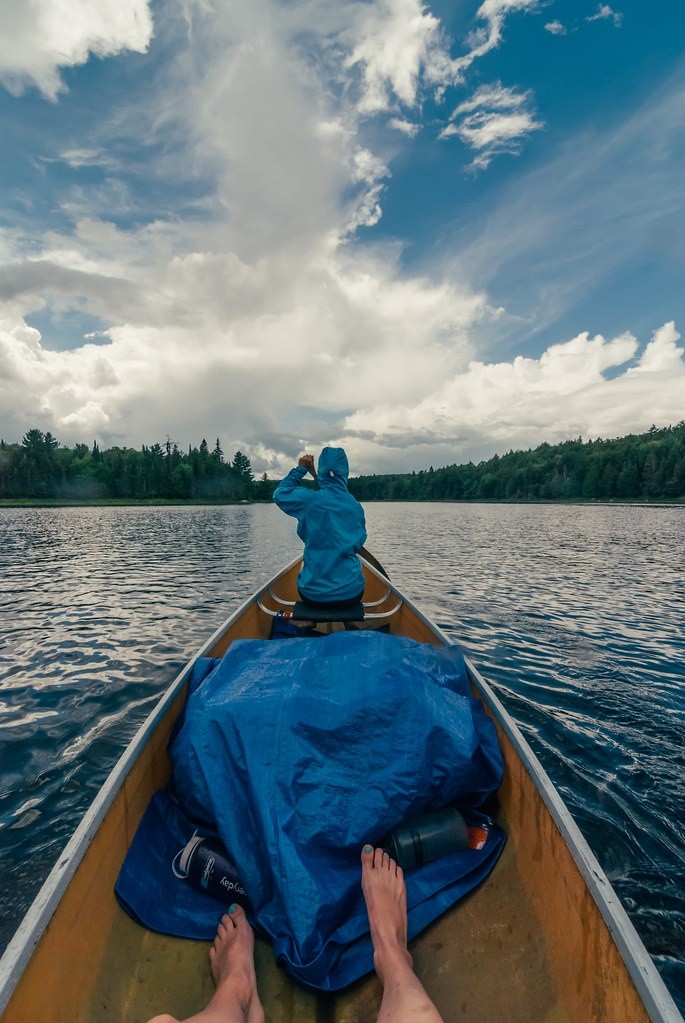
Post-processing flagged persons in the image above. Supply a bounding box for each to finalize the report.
[272,448,366,630]
[150,845,446,1023]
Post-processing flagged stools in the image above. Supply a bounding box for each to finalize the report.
[256,586,402,623]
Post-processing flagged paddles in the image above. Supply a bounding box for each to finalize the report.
[307,454,393,584]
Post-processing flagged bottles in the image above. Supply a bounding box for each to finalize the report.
[377,808,468,868]
[170,835,253,916]
